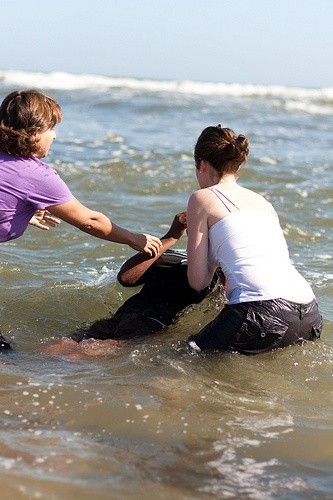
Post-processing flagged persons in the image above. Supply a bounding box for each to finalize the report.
[186,125,324,355]
[0,90,163,257]
[49,212,225,360]
